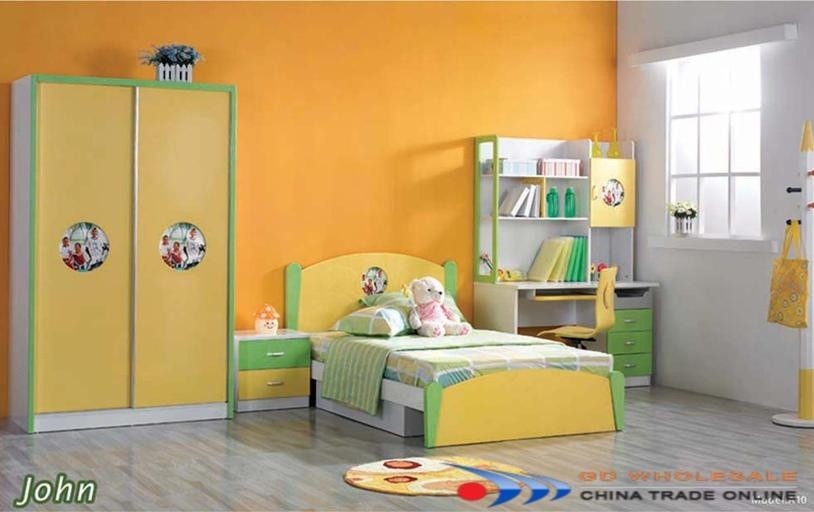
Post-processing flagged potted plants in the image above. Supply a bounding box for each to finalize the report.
[664,198,697,235]
[140,43,204,82]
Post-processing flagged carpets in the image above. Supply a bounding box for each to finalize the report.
[344,457,530,496]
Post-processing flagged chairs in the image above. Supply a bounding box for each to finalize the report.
[537,267,617,349]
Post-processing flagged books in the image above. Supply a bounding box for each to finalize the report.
[499,183,541,218]
[524,235,588,284]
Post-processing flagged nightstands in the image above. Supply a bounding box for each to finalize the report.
[234,328,312,412]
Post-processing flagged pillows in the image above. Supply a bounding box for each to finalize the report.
[327,298,411,337]
[358,292,466,322]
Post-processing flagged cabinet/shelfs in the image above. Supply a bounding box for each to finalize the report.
[9,73,236,436]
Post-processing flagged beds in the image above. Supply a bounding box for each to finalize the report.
[285,253,625,448]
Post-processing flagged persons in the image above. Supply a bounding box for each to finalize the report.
[374,271,384,293]
[65,242,88,271]
[366,279,375,294]
[361,274,368,292]
[182,227,206,267]
[160,235,171,260]
[84,227,109,267]
[601,181,623,207]
[167,242,185,269]
[60,237,73,268]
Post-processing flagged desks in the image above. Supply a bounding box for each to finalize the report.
[474,135,661,388]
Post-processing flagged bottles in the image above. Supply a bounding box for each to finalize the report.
[546,186,560,218]
[564,186,576,217]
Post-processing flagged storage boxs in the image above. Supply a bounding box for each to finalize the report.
[485,159,538,176]
[538,159,582,176]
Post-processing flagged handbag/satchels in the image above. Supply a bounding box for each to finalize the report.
[767,220,809,328]
